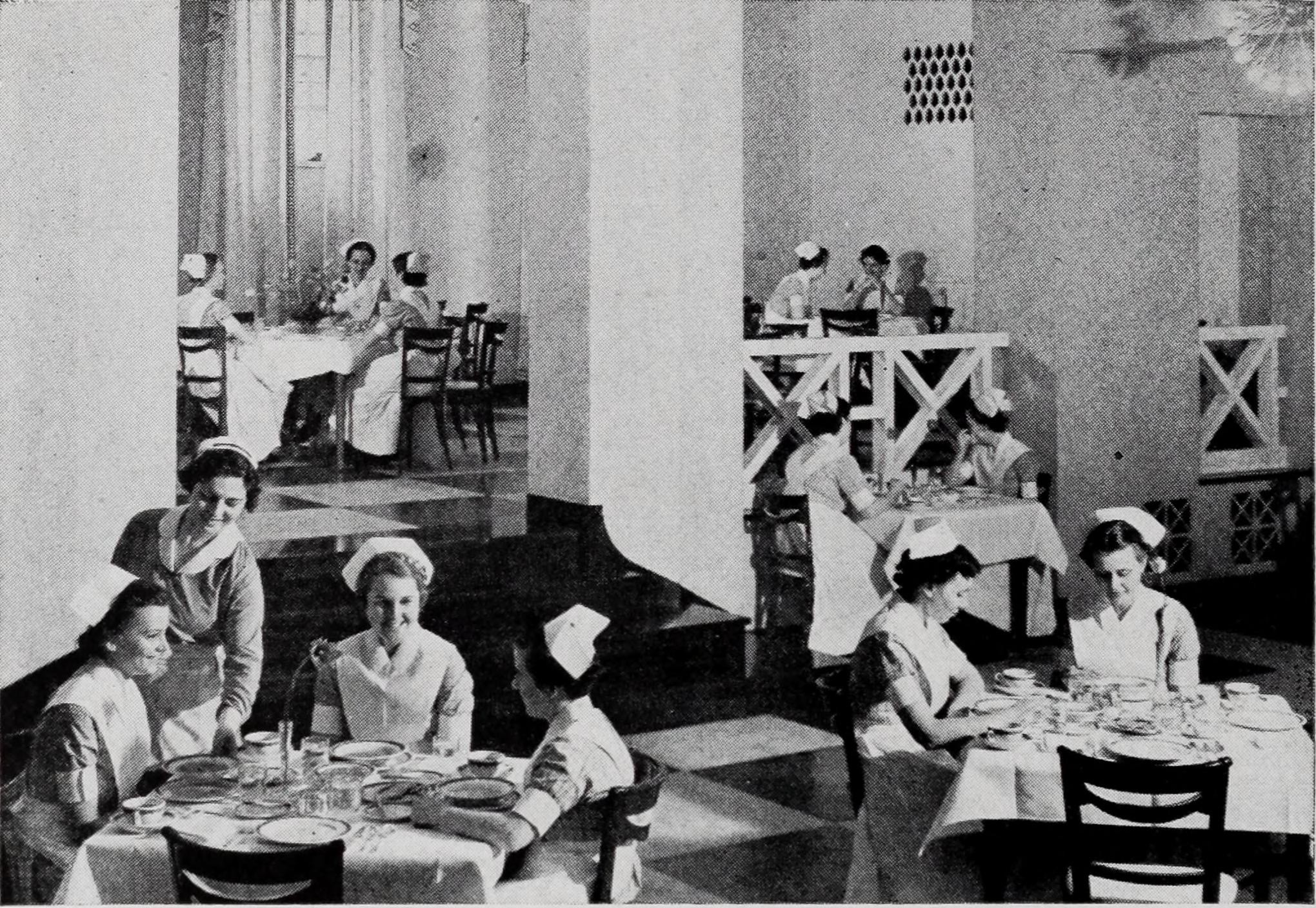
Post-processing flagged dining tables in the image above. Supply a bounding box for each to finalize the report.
[46,742,528,905]
[915,685,1316,908]
[222,324,399,474]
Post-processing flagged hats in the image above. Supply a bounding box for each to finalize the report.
[342,536,434,593]
[407,253,432,274]
[794,242,820,260]
[975,388,1006,417]
[70,565,139,626]
[909,522,957,560]
[543,604,612,680]
[807,391,838,415]
[197,436,259,471]
[180,254,207,278]
[1096,506,1166,550]
[339,239,377,256]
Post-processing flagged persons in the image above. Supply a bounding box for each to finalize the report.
[844,245,905,316]
[297,239,445,458]
[109,434,267,769]
[1049,505,1203,694]
[9,563,170,906]
[760,240,872,392]
[411,605,645,905]
[310,537,476,754]
[178,252,294,462]
[784,388,896,653]
[945,385,1057,637]
[843,515,1062,903]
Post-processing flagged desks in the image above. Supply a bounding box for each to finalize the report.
[867,478,1069,662]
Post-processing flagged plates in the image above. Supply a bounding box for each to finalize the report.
[109,734,520,846]
[974,667,1308,806]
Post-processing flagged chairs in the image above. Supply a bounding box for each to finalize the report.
[178,297,508,471]
[750,488,811,653]
[161,824,345,905]
[738,287,955,433]
[1057,746,1234,907]
[590,747,663,904]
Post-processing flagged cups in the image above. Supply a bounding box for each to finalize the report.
[120,796,166,825]
[994,668,1037,690]
[302,737,330,784]
[1061,702,1101,727]
[466,750,507,777]
[236,761,268,805]
[984,722,1024,750]
[243,732,283,759]
[326,767,363,811]
[1224,681,1260,705]
